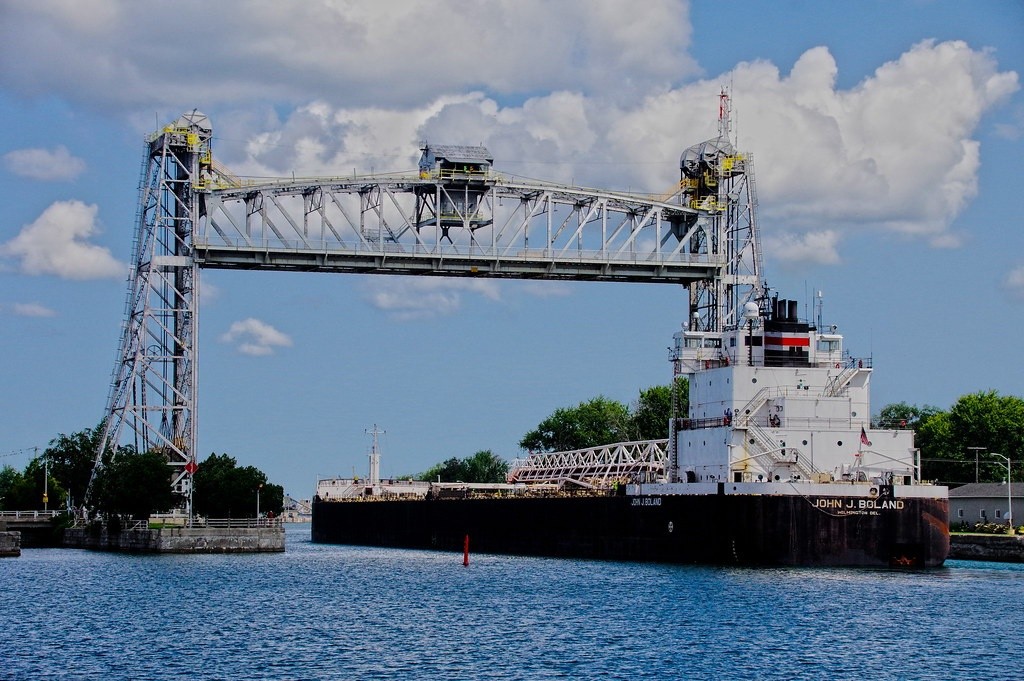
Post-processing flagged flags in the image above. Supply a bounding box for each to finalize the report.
[862,428,868,445]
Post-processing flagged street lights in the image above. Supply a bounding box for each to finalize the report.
[257,484,263,524]
[994,462,1008,470]
[990,453,1012,529]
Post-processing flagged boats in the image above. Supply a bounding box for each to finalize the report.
[311,281,951,569]
